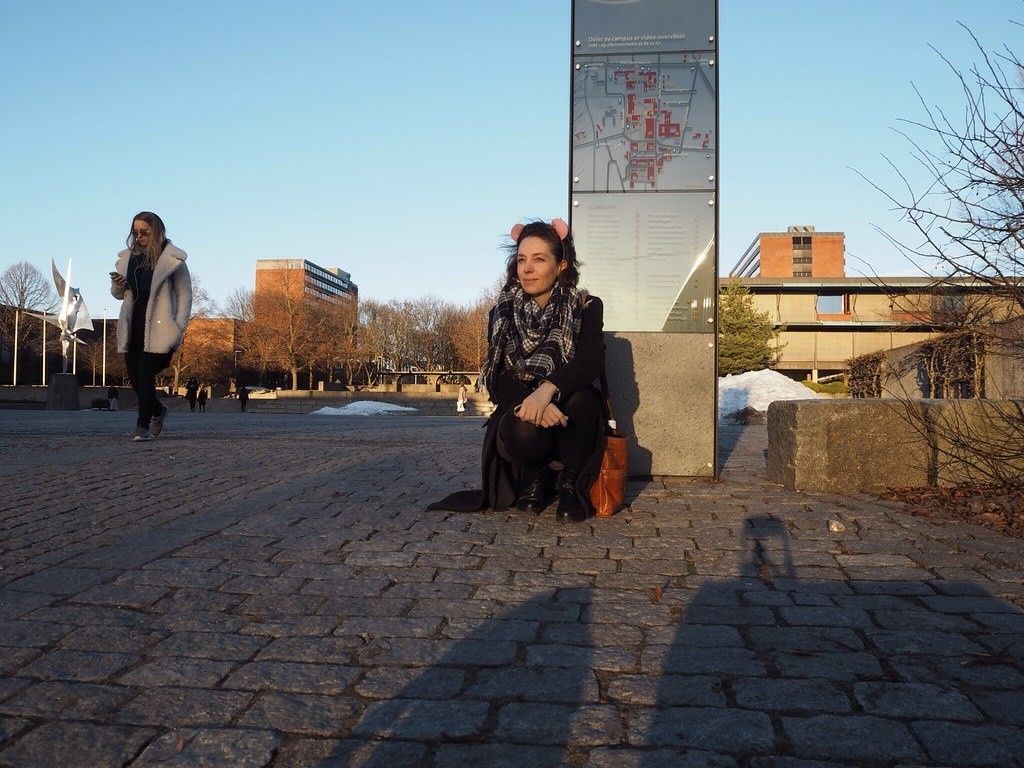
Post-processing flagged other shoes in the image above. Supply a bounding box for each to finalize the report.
[150,406,169,437]
[459,413,463,416]
[131,427,151,442]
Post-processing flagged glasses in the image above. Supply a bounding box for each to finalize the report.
[133,230,146,236]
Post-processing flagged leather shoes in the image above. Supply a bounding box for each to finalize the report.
[516,467,545,514]
[557,472,585,524]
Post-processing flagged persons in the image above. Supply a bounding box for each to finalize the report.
[456,379,468,417]
[424,216,609,523]
[185,377,198,412]
[473,377,481,394]
[196,388,206,412]
[239,387,249,412]
[110,211,193,441]
[108,384,118,411]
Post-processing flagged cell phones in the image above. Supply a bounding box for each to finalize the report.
[109,272,120,279]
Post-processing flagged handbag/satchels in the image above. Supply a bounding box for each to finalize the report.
[457,402,465,411]
[589,429,629,518]
[185,394,191,400]
